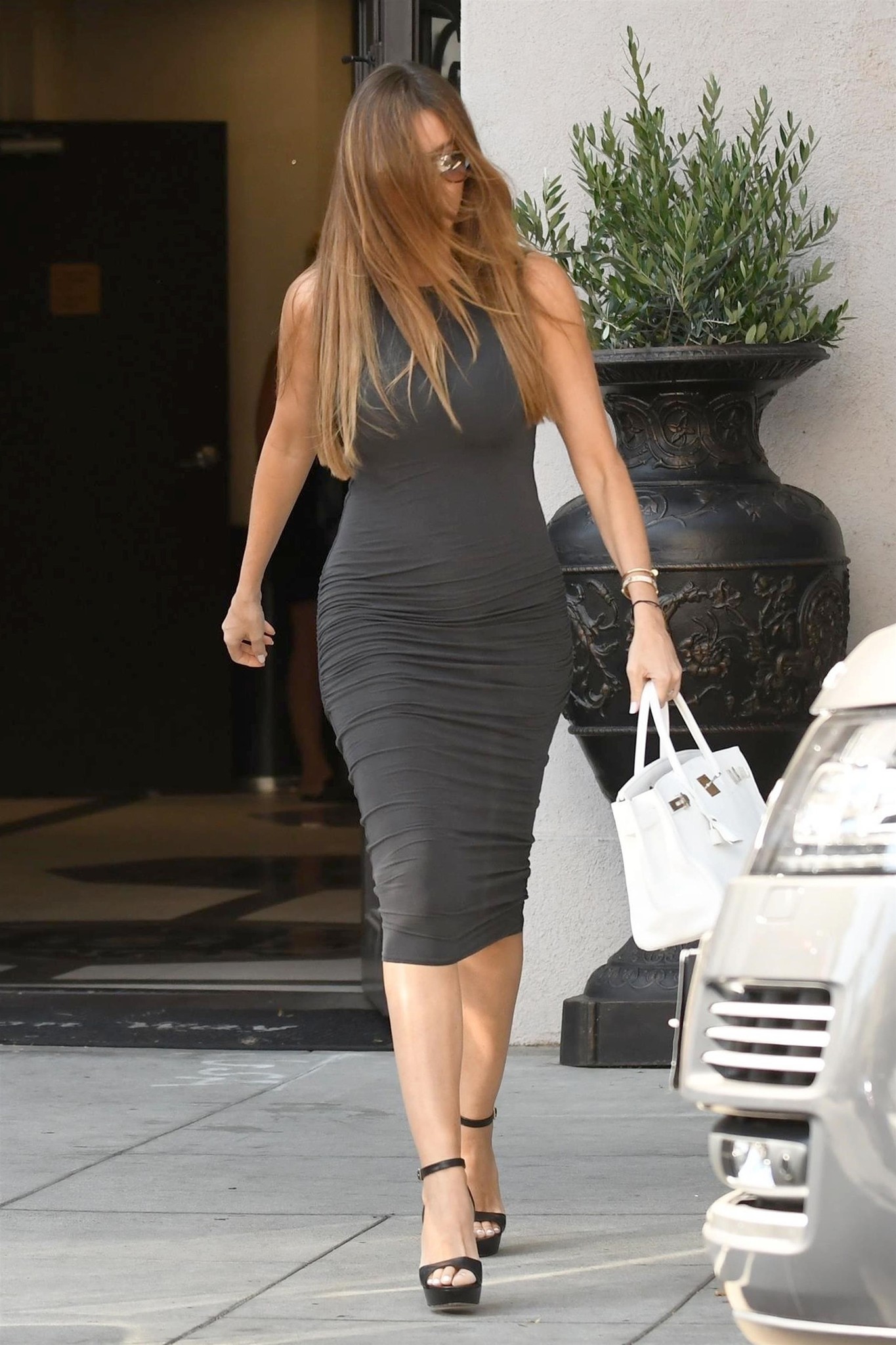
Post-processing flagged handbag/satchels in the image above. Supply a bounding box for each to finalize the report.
[612,679,768,950]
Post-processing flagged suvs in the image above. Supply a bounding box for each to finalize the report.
[671,618,896,1345]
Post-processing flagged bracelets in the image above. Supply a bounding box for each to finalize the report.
[619,569,665,622]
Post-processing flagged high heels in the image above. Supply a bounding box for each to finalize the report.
[456,1108,505,1257]
[417,1159,483,1312]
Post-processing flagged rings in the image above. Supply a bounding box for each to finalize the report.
[668,691,674,695]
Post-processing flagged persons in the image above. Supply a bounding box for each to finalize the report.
[258,238,346,800]
[221,63,684,1312]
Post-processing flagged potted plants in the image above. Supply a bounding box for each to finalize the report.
[513,21,857,1066]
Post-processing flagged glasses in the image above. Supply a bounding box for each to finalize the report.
[389,151,471,184]
